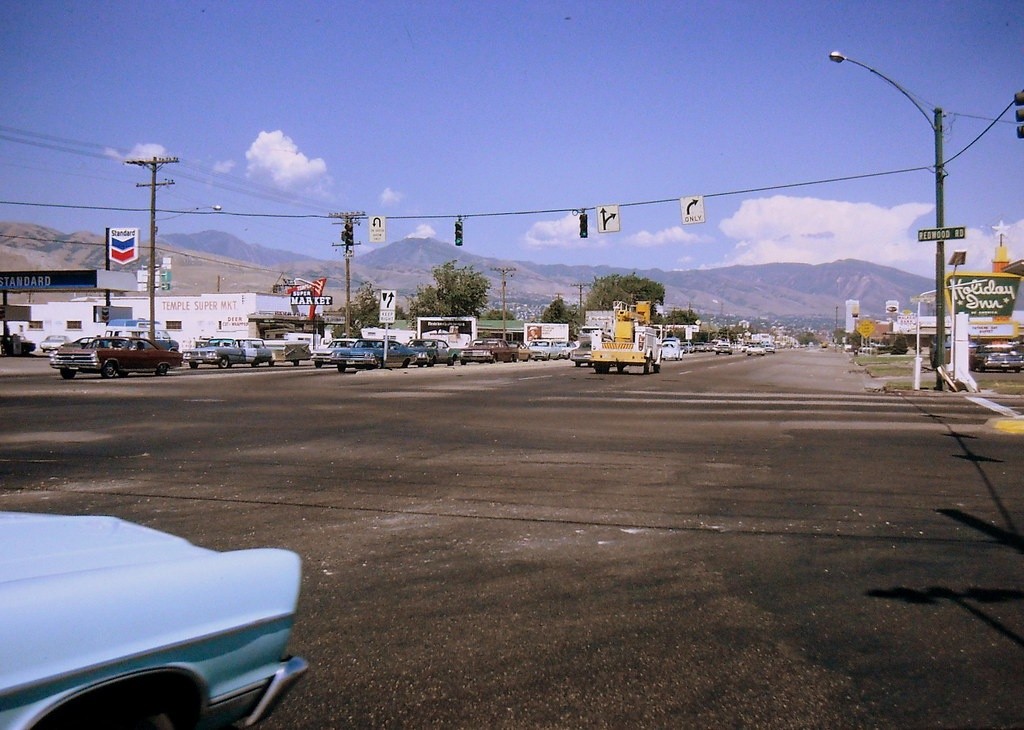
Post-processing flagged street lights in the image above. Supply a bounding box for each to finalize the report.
[149,204,222,348]
[914,277,990,392]
[827,50,950,392]
[948,250,970,382]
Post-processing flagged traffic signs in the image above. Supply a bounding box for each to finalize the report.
[680,195,705,225]
[368,216,386,243]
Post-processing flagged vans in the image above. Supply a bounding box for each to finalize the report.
[103,317,180,353]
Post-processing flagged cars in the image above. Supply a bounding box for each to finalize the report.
[182,337,247,370]
[0,334,37,357]
[308,337,363,369]
[0,506,311,730]
[236,338,273,367]
[404,338,459,368]
[39,335,72,354]
[48,334,185,379]
[329,337,420,373]
[929,332,1024,374]
[457,337,520,365]
[508,301,778,376]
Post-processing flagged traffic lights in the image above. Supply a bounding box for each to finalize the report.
[344,222,353,246]
[1013,89,1024,139]
[579,213,588,238]
[454,221,464,246]
